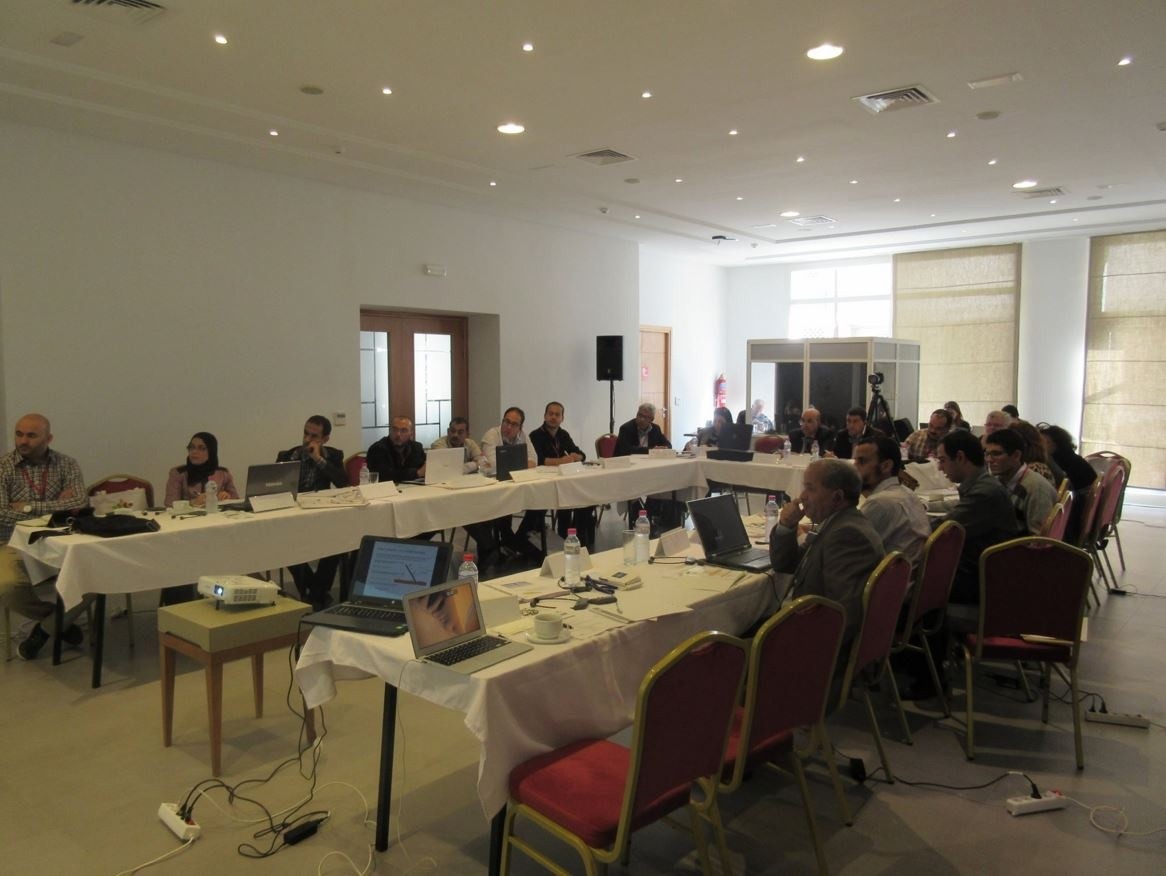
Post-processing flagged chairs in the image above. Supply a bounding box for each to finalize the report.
[343,449,376,485]
[498,623,742,876]
[831,550,915,783]
[687,595,854,876]
[888,519,966,730]
[1043,444,1129,610]
[967,534,1090,775]
[595,436,620,458]
[85,475,157,649]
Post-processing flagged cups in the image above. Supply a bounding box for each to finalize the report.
[173,500,189,511]
[533,613,570,638]
[370,472,379,483]
[623,529,637,564]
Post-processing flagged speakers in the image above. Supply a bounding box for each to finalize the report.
[595,335,624,381]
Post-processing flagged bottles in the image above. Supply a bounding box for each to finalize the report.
[691,434,698,455]
[765,495,779,541]
[565,527,583,585]
[479,450,488,476]
[785,438,791,464]
[460,554,478,593]
[359,463,370,485]
[752,415,768,432]
[635,510,651,562]
[811,440,819,461]
[206,476,219,513]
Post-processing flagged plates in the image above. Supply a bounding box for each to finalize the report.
[527,632,573,644]
[168,508,195,514]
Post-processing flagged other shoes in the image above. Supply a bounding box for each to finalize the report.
[61,624,83,646]
[301,593,334,611]
[16,623,50,660]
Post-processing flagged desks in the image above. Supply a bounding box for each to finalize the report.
[159,596,316,771]
[9,437,959,876]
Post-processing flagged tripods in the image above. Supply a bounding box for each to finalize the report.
[864,386,899,442]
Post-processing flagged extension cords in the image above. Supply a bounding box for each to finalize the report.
[1007,791,1069,818]
[157,802,200,841]
[1084,708,1150,728]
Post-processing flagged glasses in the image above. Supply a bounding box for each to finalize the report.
[503,418,521,428]
[186,444,207,451]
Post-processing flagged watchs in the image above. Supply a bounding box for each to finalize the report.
[22,501,34,515]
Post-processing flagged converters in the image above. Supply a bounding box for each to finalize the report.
[283,821,318,844]
[1111,588,1126,595]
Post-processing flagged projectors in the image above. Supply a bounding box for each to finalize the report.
[196,572,277,606]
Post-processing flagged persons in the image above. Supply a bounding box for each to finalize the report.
[703,407,734,445]
[764,459,890,707]
[846,433,937,636]
[977,410,1010,441]
[431,416,508,576]
[484,401,540,564]
[1038,419,1097,487]
[2,410,96,663]
[984,429,1060,535]
[903,407,951,459]
[167,430,245,502]
[274,414,354,609]
[1002,404,1028,423]
[788,407,834,457]
[528,400,589,551]
[943,399,971,435]
[829,406,882,458]
[370,416,430,485]
[613,401,674,529]
[924,425,1022,607]
[1009,419,1057,491]
[736,398,774,434]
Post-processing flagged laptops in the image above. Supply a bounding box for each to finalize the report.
[717,422,753,449]
[218,460,302,511]
[406,577,532,677]
[301,535,452,639]
[489,442,528,482]
[401,446,464,487]
[688,493,772,572]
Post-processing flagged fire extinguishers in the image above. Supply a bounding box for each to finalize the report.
[715,373,726,408]
